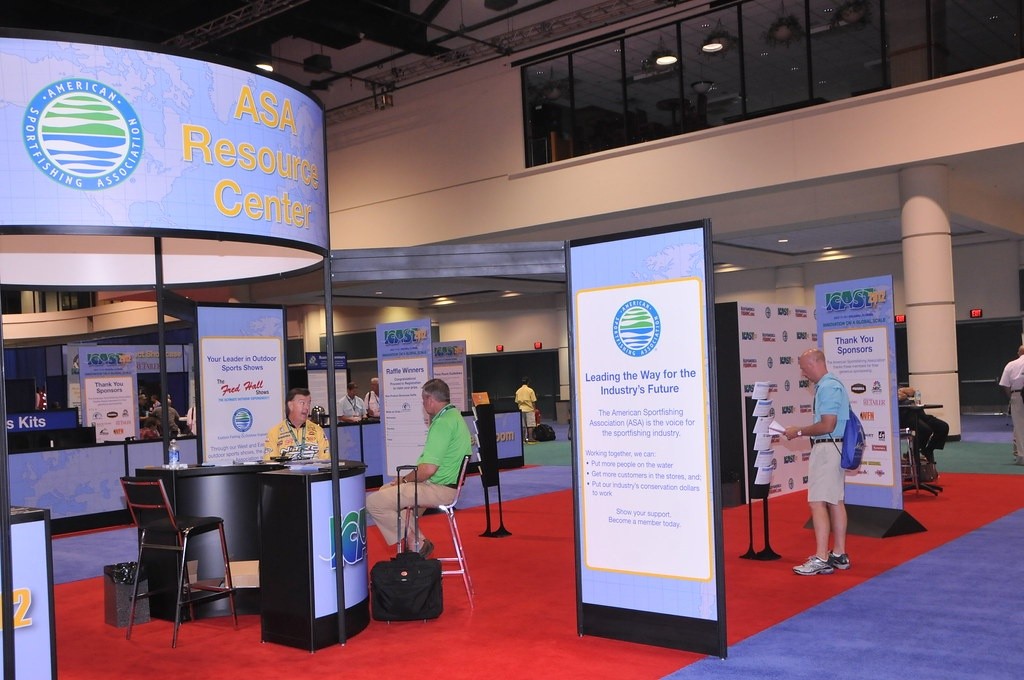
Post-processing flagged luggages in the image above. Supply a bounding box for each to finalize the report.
[369,465,444,622]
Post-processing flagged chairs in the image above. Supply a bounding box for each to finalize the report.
[634,94,709,142]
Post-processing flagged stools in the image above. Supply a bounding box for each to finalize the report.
[120,475,237,648]
[899,428,918,495]
[405,455,474,606]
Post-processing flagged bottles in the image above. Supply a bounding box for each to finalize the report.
[168,439,180,465]
[914,389,921,405]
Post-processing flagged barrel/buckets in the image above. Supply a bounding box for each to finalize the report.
[311,405,325,425]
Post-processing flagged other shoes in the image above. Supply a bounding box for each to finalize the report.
[419,538,435,556]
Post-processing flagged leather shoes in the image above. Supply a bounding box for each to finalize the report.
[921,447,937,464]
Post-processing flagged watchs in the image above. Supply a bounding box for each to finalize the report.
[402,475,407,483]
[796,427,803,437]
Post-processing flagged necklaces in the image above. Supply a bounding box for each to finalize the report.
[346,395,356,412]
[374,393,380,406]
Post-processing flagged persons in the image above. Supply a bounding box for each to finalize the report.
[897,384,949,466]
[515,376,539,444]
[364,377,381,417]
[138,394,180,440]
[187,405,197,439]
[999,344,1024,466]
[366,380,472,558]
[782,348,851,575]
[262,388,330,460]
[337,381,368,424]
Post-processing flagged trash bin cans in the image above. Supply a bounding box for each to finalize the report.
[104,562,150,627]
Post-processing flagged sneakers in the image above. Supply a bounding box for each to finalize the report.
[828,550,850,570]
[792,554,834,574]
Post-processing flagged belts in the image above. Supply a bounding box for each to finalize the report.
[1012,389,1021,394]
[447,483,459,489]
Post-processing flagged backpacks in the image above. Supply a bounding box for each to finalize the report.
[817,378,865,470]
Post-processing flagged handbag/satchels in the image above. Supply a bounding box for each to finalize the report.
[531,424,556,442]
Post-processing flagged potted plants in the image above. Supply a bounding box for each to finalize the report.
[641,47,678,73]
[829,0,877,32]
[540,76,569,102]
[697,30,738,59]
[761,13,804,48]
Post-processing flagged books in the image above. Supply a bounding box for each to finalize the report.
[752,380,774,484]
[285,460,345,469]
[769,419,785,436]
[235,459,280,465]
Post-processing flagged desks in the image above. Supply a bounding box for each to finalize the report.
[657,97,690,128]
[900,404,944,495]
